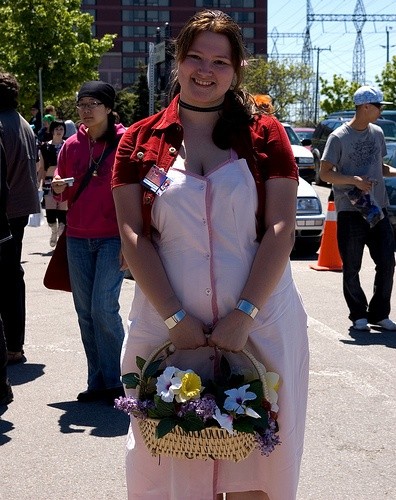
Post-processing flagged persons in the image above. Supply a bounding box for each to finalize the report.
[319,86,396,330]
[50,81,129,408]
[0,73,47,417]
[30,103,77,247]
[114,11,310,500]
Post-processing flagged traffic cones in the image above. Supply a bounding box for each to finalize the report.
[310,200,344,271]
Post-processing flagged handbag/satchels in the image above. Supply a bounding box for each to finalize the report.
[43,225,72,292]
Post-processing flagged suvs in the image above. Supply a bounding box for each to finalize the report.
[313,110,396,214]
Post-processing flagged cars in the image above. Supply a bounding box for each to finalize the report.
[294,175,326,238]
[280,122,315,172]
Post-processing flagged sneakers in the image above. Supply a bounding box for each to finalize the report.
[352,318,370,331]
[374,318,396,331]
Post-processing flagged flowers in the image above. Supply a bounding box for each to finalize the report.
[111,356,281,457]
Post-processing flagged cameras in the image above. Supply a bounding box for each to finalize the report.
[61,177,75,187]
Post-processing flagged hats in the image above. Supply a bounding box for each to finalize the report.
[353,86,393,105]
[77,81,115,111]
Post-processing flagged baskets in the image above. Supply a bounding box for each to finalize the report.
[136,333,269,462]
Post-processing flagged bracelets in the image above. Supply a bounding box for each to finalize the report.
[234,299,259,320]
[164,308,187,330]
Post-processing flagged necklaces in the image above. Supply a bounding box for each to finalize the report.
[176,98,224,112]
[87,130,108,176]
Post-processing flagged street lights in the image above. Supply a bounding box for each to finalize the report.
[308,48,332,125]
[380,44,396,62]
[39,67,45,129]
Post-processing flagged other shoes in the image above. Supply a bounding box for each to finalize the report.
[77,385,126,405]
[7,350,22,363]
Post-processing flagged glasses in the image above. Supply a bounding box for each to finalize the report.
[76,101,103,110]
[369,103,386,111]
